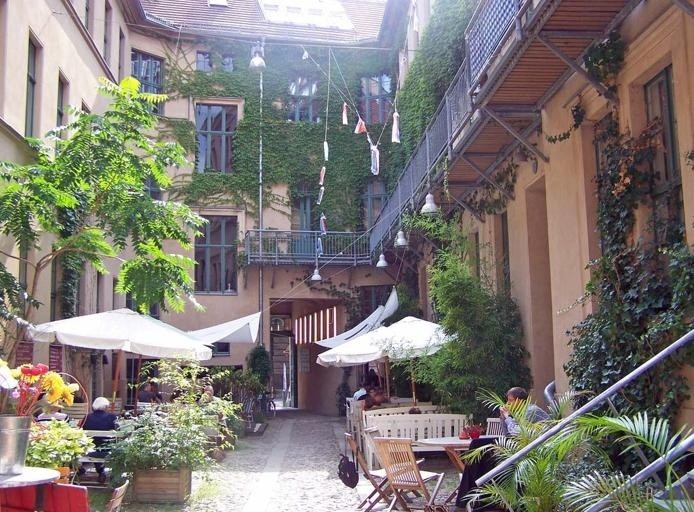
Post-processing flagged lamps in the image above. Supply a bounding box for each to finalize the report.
[246,46,267,72]
[420,129,438,218]
[310,233,322,281]
[375,179,406,268]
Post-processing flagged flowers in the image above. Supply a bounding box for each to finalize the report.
[9,359,79,419]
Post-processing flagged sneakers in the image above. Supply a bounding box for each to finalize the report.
[75,466,106,482]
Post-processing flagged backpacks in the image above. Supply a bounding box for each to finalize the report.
[337,433,358,488]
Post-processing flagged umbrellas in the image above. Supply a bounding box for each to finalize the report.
[316,324,392,397]
[27,305,215,414]
[314,313,459,406]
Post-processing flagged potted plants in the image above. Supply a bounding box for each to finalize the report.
[110,413,204,503]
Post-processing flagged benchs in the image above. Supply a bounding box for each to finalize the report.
[344,396,474,470]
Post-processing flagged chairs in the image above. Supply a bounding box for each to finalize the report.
[340,426,445,512]
[483,417,508,438]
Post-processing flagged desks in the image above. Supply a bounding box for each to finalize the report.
[0,464,61,511]
[418,433,505,491]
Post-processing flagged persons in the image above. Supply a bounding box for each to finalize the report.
[365,392,387,411]
[35,393,85,478]
[447,392,607,512]
[353,381,367,400]
[368,381,377,392]
[356,388,376,401]
[138,377,213,408]
[82,396,124,486]
[367,368,380,386]
[467,386,555,460]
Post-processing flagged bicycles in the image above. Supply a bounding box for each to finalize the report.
[252,390,277,423]
